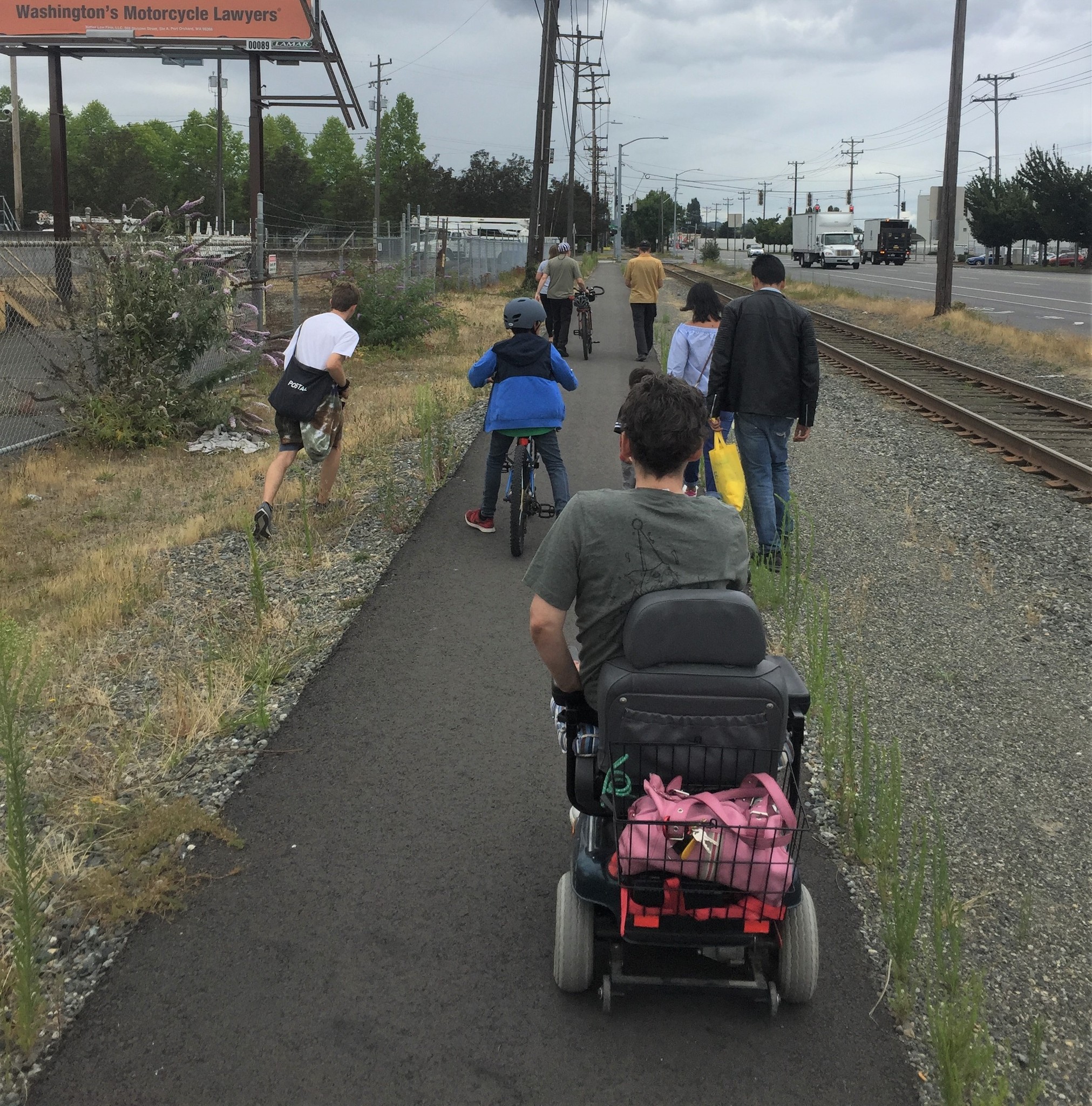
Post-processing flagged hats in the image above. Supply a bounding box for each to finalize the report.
[639,240,651,248]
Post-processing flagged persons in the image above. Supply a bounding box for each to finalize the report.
[536,245,560,343]
[667,281,736,501]
[252,281,361,542]
[613,368,655,489]
[706,253,820,572]
[534,242,590,357]
[523,373,752,734]
[623,240,666,361]
[464,297,579,533]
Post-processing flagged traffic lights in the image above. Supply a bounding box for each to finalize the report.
[806,193,813,207]
[814,206,819,212]
[757,191,764,206]
[788,207,793,217]
[901,201,906,211]
[849,206,854,214]
[846,191,852,205]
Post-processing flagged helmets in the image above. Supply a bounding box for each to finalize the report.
[504,297,547,330]
[557,242,570,252]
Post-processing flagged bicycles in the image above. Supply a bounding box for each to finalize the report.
[573,286,605,361]
[479,370,578,558]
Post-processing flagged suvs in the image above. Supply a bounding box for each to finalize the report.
[1030,251,1056,265]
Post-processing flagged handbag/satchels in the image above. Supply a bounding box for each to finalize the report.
[268,354,332,423]
[708,418,745,512]
[618,772,794,908]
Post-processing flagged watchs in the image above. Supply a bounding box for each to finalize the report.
[338,378,350,391]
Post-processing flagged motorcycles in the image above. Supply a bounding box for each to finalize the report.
[547,587,822,1006]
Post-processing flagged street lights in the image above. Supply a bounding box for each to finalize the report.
[566,121,623,257]
[673,169,704,258]
[928,219,933,253]
[616,136,669,262]
[876,172,900,219]
[368,94,388,264]
[197,72,228,234]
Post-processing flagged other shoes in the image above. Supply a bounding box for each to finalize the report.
[548,338,553,343]
[782,541,794,555]
[683,484,698,497]
[750,550,782,572]
[636,354,646,361]
[252,501,273,541]
[553,343,569,357]
[314,499,336,519]
[465,508,496,533]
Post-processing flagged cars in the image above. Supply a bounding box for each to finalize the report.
[965,253,1002,266]
[680,245,685,249]
[747,245,764,258]
[1048,252,1085,268]
[687,245,694,250]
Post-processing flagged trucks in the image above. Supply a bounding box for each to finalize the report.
[410,228,520,265]
[791,212,861,270]
[855,219,912,266]
